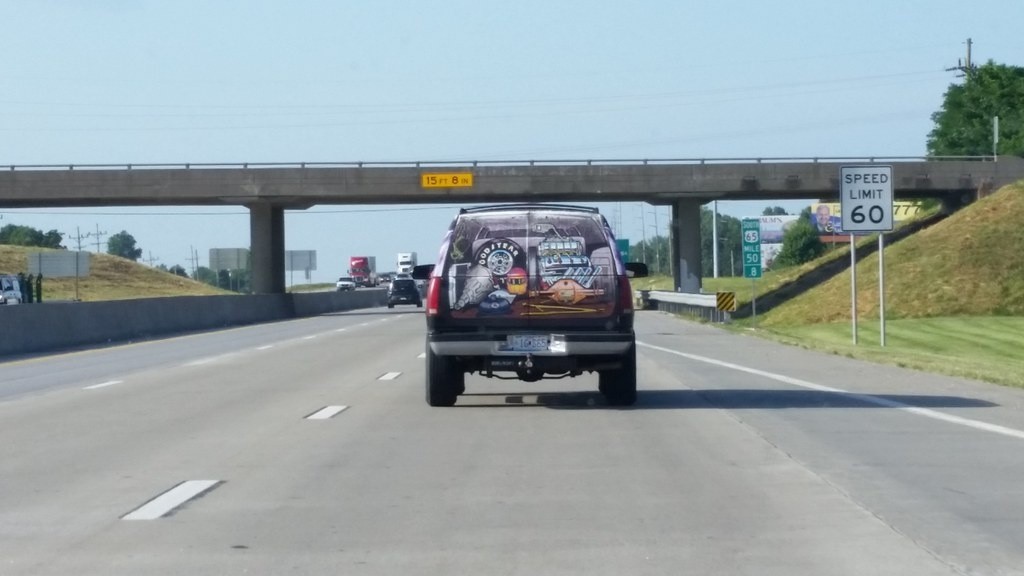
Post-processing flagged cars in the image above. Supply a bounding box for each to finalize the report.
[412,264,436,297]
[377,272,391,283]
[336,275,356,291]
[0,269,22,307]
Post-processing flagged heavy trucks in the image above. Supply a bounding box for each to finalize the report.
[397,250,418,276]
[346,256,380,288]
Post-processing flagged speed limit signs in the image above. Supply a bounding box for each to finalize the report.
[839,161,894,234]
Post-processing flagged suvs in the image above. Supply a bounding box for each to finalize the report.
[425,202,648,409]
[385,277,424,309]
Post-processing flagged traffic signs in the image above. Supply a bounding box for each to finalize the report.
[742,218,762,279]
